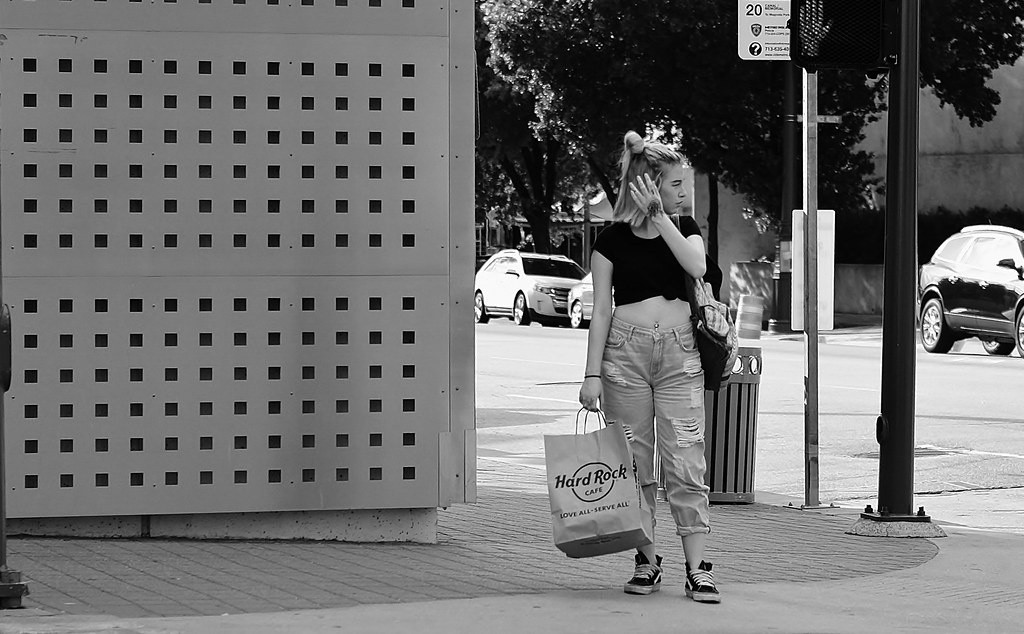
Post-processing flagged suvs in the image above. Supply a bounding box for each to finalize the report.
[475,249,589,326]
[915,223,1024,361]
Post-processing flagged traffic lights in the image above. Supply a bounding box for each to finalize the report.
[785,0,834,76]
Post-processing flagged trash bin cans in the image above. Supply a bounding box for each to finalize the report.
[726,260,774,331]
[703,347,762,505]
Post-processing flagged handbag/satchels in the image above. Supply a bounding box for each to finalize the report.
[544,406,653,558]
[672,213,739,390]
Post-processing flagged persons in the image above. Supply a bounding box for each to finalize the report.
[579,131,722,603]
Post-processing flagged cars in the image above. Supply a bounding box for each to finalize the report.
[566,264,615,329]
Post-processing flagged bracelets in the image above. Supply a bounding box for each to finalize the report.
[584,375,601,379]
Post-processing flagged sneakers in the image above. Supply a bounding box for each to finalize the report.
[684,560,721,601]
[624,549,663,594]
